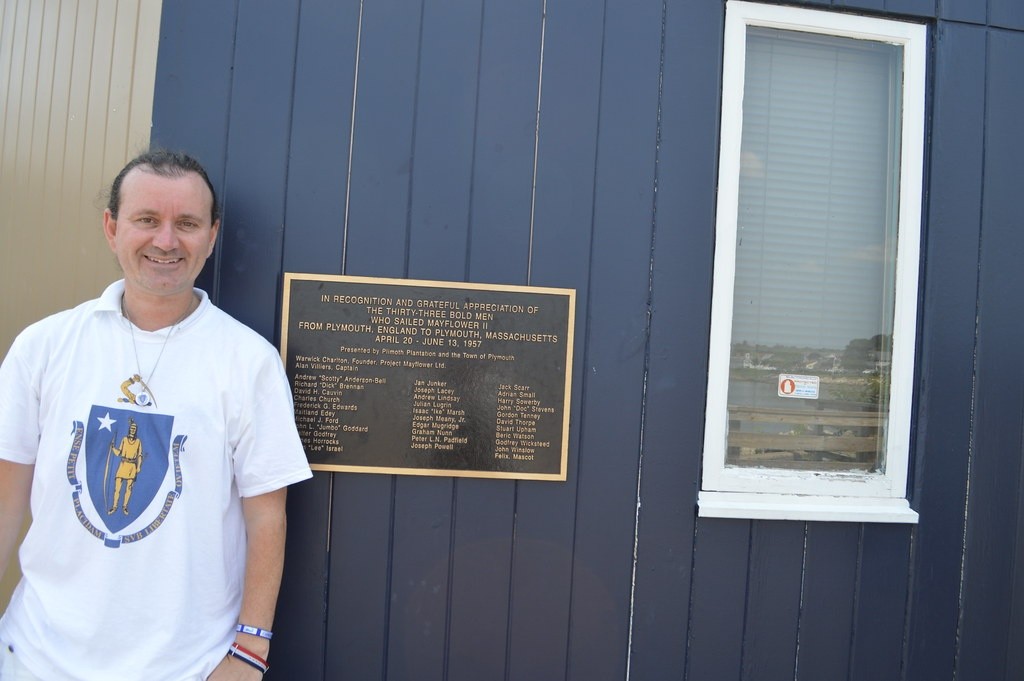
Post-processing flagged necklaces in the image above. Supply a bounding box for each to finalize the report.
[122,296,194,407]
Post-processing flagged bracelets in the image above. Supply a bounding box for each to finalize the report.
[237,624,273,639]
[227,643,269,674]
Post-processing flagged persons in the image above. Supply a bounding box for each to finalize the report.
[0,150,313,681]
